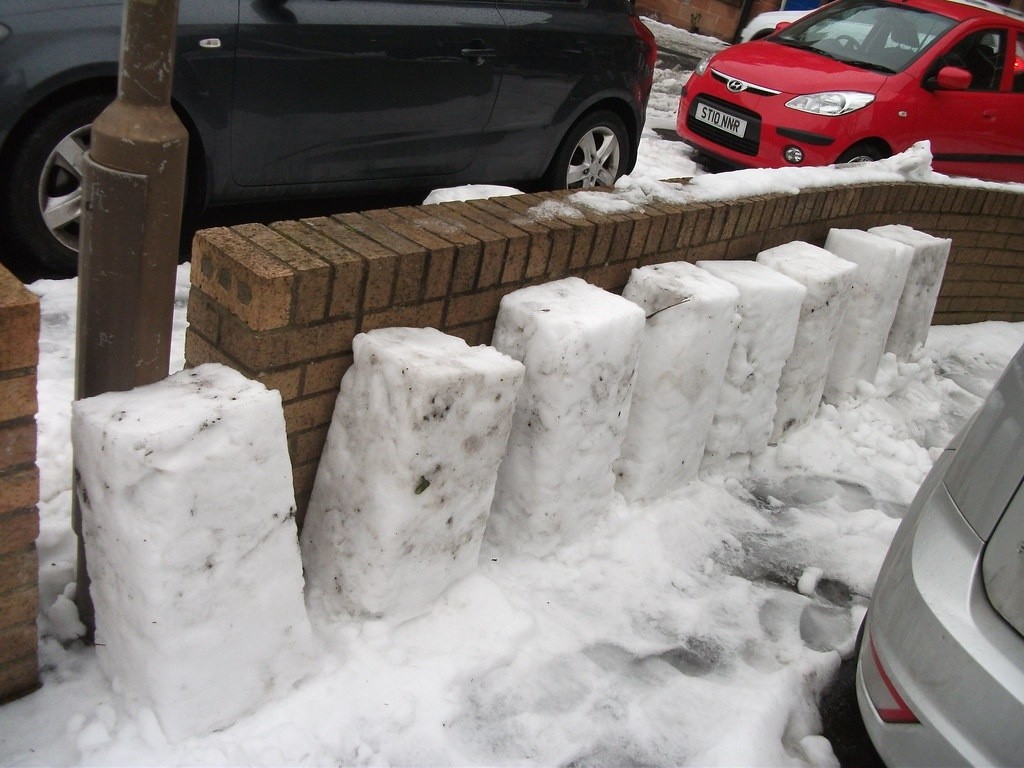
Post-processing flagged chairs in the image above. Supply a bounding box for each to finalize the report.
[872,18,920,72]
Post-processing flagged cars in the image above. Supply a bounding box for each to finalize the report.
[737,1,1022,80]
[2,0,655,287]
[672,0,1023,198]
